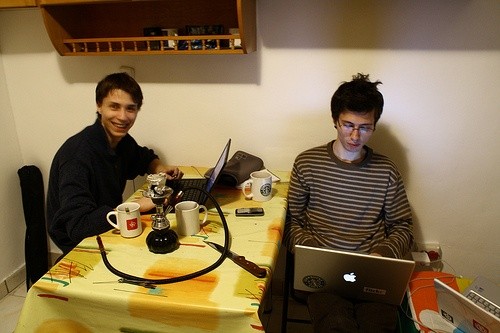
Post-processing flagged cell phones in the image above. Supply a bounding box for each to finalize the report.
[235,208,264,216]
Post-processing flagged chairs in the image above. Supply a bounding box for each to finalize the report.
[18,164,64,293]
[282,249,313,333]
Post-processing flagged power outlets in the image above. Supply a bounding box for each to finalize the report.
[414,241,441,262]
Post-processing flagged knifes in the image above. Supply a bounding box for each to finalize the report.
[204,240,266,278]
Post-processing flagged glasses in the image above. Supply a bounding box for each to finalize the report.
[338,118,376,134]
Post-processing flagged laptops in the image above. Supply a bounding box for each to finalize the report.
[433,275,500,333]
[140,138,231,215]
[292,245,415,306]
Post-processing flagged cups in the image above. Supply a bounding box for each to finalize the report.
[185,25,205,50]
[228,28,241,48]
[205,25,225,49]
[161,27,180,49]
[143,26,161,50]
[106,202,142,238]
[174,201,208,237]
[241,170,272,202]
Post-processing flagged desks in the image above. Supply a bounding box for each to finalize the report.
[14,166,292,333]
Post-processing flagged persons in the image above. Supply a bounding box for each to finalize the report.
[282,74,414,333]
[46,73,184,254]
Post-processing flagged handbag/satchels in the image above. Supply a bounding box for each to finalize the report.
[204,151,262,186]
